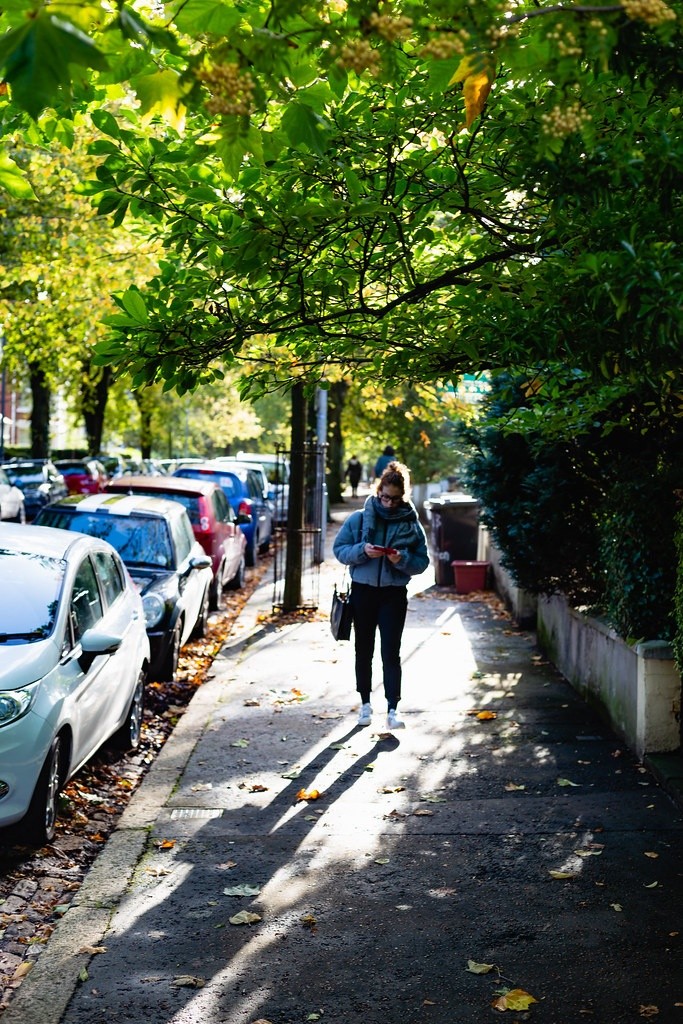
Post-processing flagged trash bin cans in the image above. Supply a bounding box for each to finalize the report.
[423,495,481,586]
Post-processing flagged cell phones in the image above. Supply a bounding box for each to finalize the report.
[374,545,397,555]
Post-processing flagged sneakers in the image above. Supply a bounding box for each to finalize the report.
[386,709,406,730]
[358,703,373,726]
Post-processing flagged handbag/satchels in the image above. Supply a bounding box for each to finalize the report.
[330,590,352,641]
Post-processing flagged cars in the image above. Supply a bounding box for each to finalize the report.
[30,492,216,684]
[0,450,290,533]
[105,474,253,613]
[0,521,158,846]
[171,462,279,568]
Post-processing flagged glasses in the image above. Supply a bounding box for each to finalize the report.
[380,489,403,504]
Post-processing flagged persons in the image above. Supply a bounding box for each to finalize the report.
[344,455,363,499]
[332,461,431,730]
[375,446,399,480]
